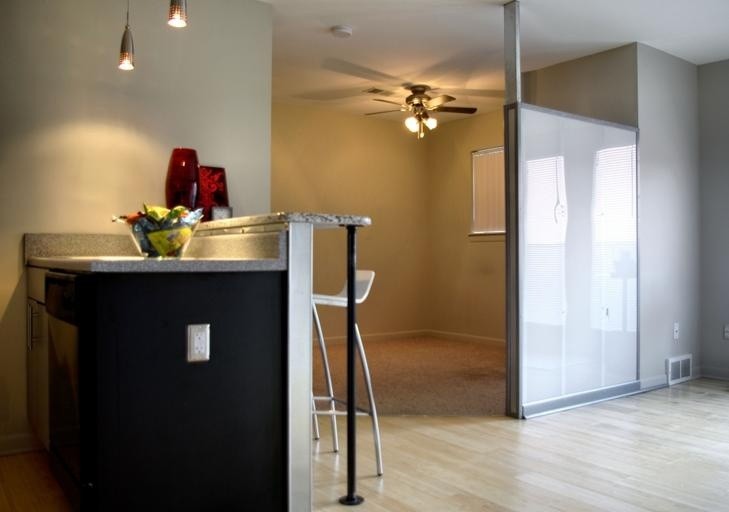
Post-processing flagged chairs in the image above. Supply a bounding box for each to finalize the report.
[313,261,396,485]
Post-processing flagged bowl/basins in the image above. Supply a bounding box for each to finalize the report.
[127,220,202,258]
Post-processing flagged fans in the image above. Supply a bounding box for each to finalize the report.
[363,83,476,143]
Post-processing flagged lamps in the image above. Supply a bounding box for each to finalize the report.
[116,41,135,70]
[163,0,194,27]
[402,93,443,139]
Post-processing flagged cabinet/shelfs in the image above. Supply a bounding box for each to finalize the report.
[24,213,343,507]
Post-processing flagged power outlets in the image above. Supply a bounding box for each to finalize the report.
[672,321,682,343]
[184,323,214,363]
[724,326,728,339]
